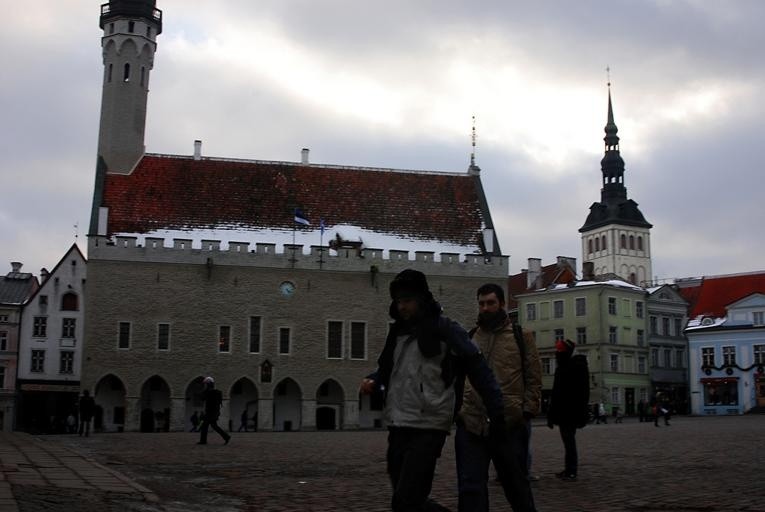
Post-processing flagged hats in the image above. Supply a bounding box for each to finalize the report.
[389,268,439,323]
[202,376,214,384]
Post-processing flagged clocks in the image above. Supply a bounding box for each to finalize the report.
[278,280,296,298]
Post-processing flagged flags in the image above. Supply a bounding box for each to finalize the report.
[293,209,311,225]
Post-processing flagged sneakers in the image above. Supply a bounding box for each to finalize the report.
[526,469,578,482]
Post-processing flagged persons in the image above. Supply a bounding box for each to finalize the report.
[545,338,592,483]
[190,411,200,432]
[589,397,673,428]
[200,409,204,432]
[360,269,507,510]
[66,411,76,434]
[236,408,251,432]
[198,375,231,444]
[78,390,96,437]
[456,282,542,509]
[251,410,257,431]
